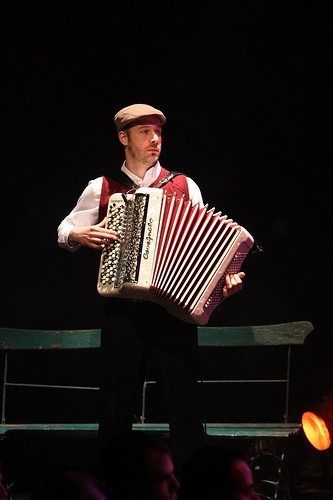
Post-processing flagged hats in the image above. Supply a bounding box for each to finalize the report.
[115,104,166,135]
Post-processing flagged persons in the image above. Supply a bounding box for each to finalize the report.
[57,103,248,492]
[183,444,261,500]
[90,427,181,500]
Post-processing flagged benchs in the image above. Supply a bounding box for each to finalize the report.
[0,321,314,499]
